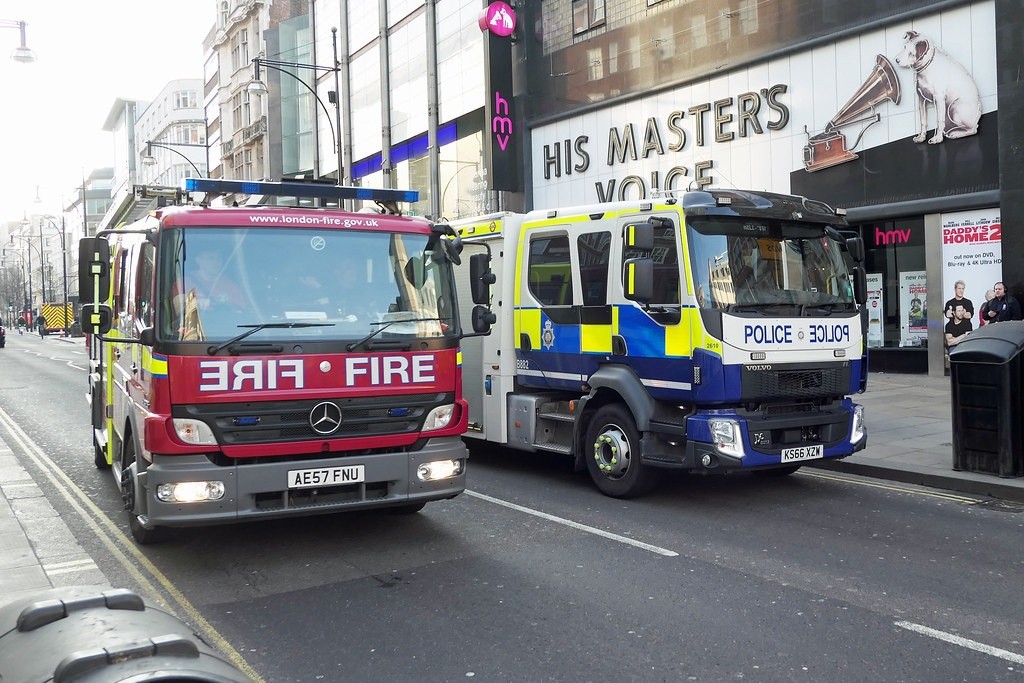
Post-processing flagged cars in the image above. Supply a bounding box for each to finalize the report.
[0,326,6,348]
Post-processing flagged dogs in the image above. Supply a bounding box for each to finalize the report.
[895,30,982,145]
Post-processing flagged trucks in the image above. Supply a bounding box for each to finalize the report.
[41,302,72,334]
[413,178,869,502]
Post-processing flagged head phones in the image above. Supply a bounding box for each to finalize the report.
[994,282,1008,293]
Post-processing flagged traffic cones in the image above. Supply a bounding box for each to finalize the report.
[58,329,65,338]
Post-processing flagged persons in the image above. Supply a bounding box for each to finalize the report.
[18,316,26,327]
[944,280,996,356]
[172,248,244,319]
[297,275,329,306]
[983,282,1022,324]
[34,312,49,335]
[908,293,928,327]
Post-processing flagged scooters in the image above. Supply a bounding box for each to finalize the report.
[19,323,26,336]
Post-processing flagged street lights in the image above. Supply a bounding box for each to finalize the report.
[18,209,69,338]
[0,238,33,333]
[246,26,345,210]
[141,115,213,207]
[9,224,46,303]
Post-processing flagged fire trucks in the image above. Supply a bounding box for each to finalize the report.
[77,178,498,545]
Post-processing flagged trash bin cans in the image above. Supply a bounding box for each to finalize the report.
[71,321,82,337]
[949,320,1024,478]
